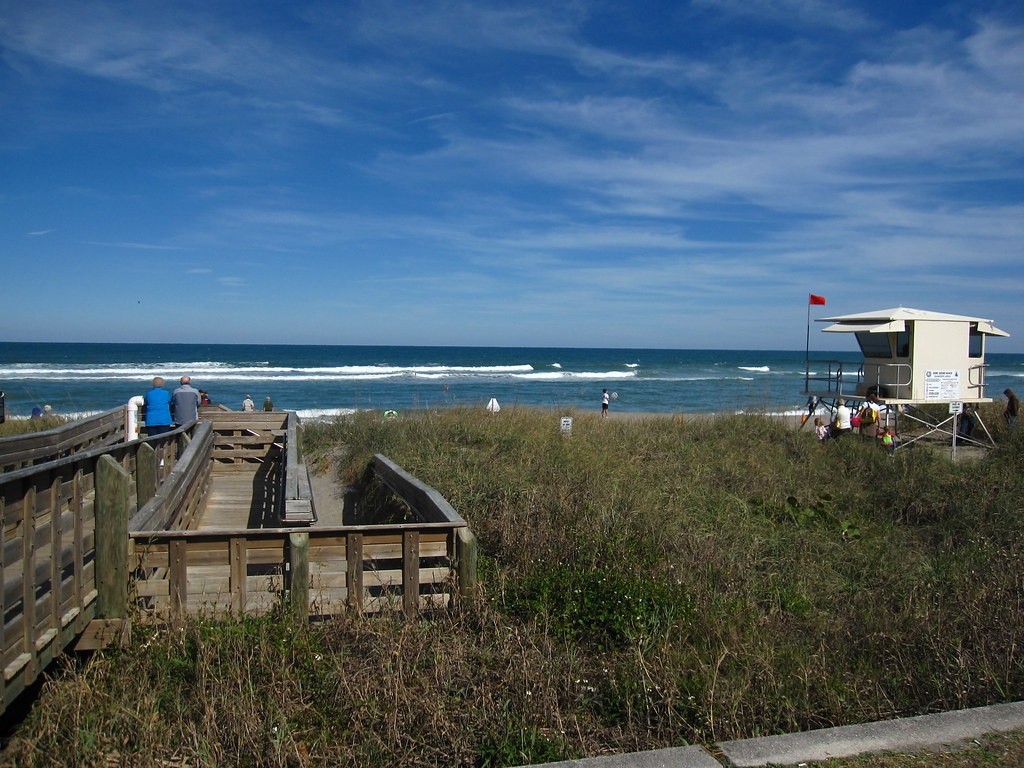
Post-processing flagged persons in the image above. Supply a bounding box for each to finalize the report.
[386,411,397,420]
[1003,388,1020,435]
[958,403,980,440]
[806,390,905,455]
[143,375,274,466]
[0,391,6,424]
[31,404,56,418]
[602,388,611,418]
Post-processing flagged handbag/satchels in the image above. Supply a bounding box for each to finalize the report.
[827,422,840,439]
[861,404,878,424]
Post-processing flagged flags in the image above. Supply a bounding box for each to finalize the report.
[810,293,825,305]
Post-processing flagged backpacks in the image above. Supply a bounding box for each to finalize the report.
[882,432,893,445]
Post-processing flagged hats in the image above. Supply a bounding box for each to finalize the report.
[45,405,52,411]
[836,399,844,405]
